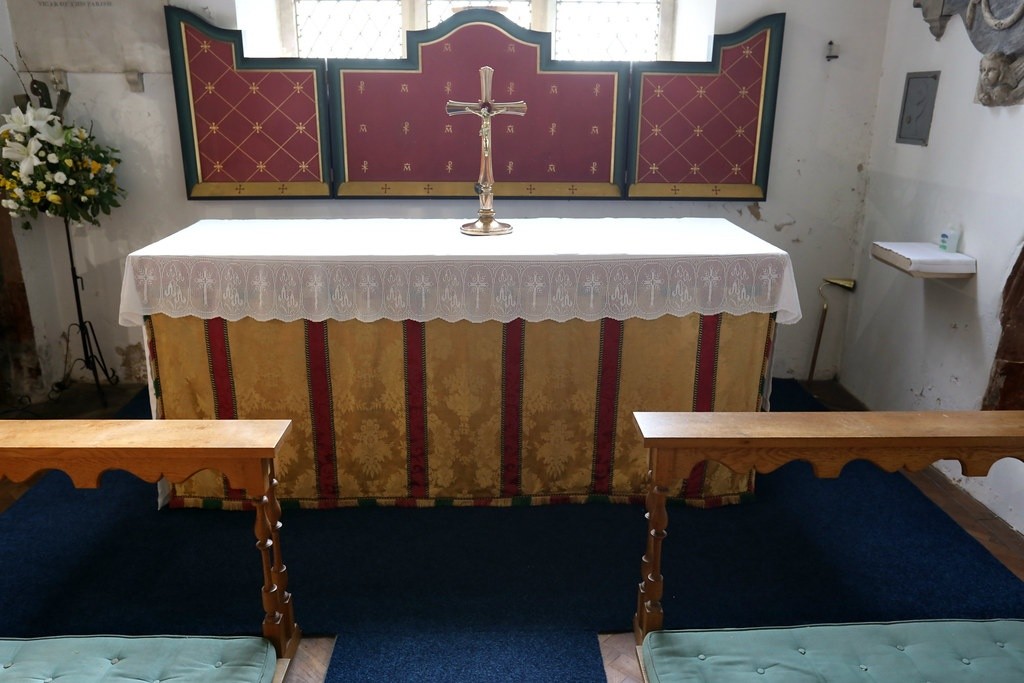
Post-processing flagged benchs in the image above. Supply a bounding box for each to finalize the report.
[0,421,300,666]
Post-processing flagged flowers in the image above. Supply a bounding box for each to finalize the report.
[0,43,127,226]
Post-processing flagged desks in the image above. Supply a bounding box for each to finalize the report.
[119,219,802,509]
[633,410,1024,659]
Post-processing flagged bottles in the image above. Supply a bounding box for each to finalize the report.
[940,206,963,252]
